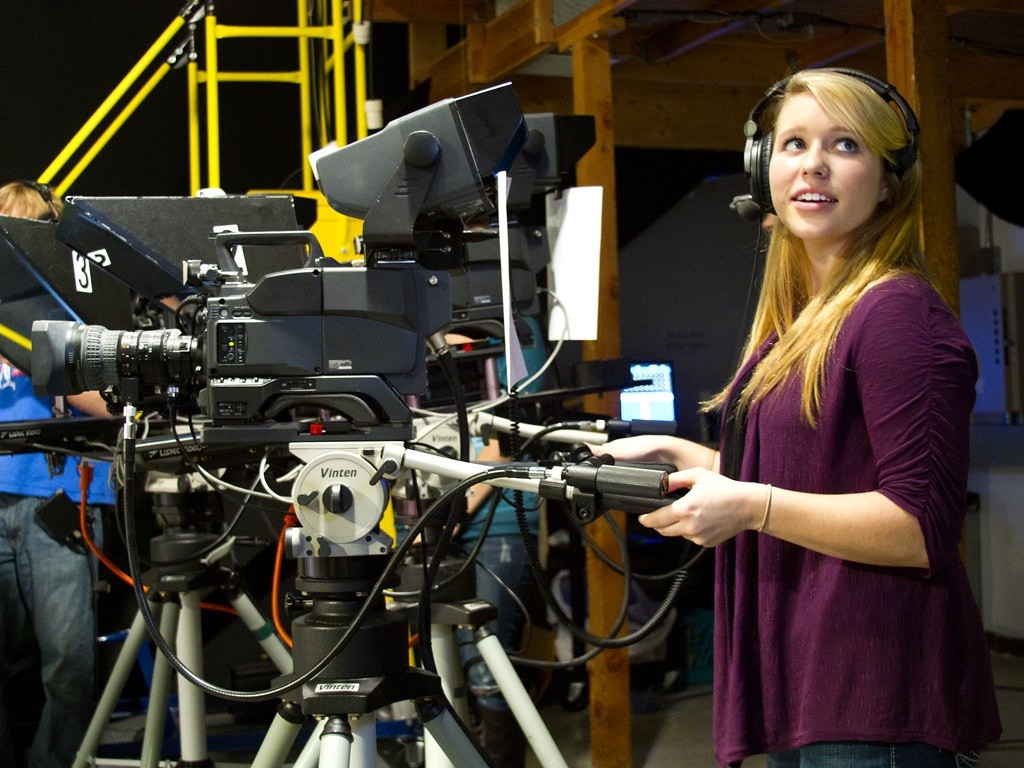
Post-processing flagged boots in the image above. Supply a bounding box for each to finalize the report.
[476,703,527,768]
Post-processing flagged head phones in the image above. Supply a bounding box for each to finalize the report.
[745,66,921,215]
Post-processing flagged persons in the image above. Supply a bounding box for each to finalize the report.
[450,320,548,652]
[587,70,1002,768]
[0,180,119,767]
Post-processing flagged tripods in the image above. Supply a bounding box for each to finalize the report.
[73,410,694,768]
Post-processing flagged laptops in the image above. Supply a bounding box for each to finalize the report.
[620,364,676,424]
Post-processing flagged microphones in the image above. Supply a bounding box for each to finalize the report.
[729,194,761,222]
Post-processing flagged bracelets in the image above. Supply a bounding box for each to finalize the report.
[758,484,772,533]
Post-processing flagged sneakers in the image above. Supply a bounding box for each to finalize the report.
[639,659,713,705]
